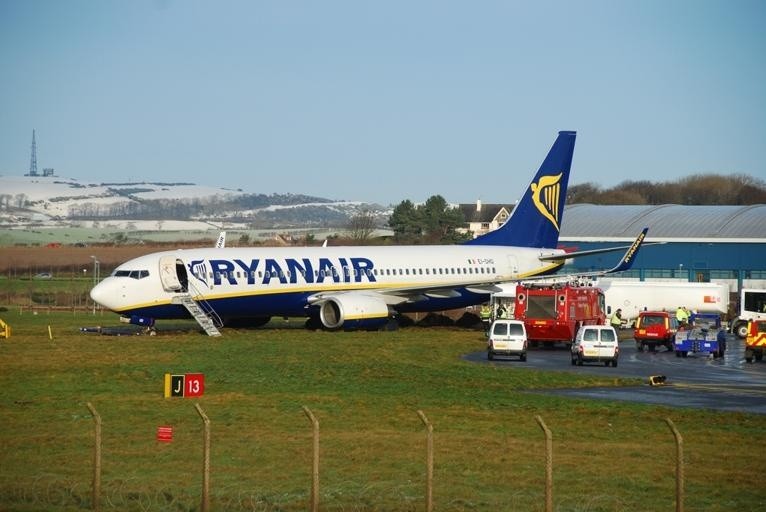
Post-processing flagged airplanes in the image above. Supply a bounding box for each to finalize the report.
[90,130,668,338]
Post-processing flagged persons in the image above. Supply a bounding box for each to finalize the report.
[480,304,493,337]
[609,308,628,342]
[496,304,507,320]
[760,301,766,312]
[683,307,690,317]
[676,307,687,322]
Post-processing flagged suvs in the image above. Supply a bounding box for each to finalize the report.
[633,311,676,352]
[485,319,528,361]
[569,325,620,367]
[745,316,766,361]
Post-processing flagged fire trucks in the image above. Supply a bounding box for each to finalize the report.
[515,276,605,347]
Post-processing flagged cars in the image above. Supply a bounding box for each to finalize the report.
[35,273,53,279]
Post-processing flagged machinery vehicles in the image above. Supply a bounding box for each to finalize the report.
[672,315,727,358]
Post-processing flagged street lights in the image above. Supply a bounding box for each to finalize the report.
[679,264,684,278]
[89,255,101,317]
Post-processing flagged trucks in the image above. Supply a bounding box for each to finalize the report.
[595,278,766,339]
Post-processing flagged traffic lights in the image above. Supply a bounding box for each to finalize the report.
[650,375,667,385]
